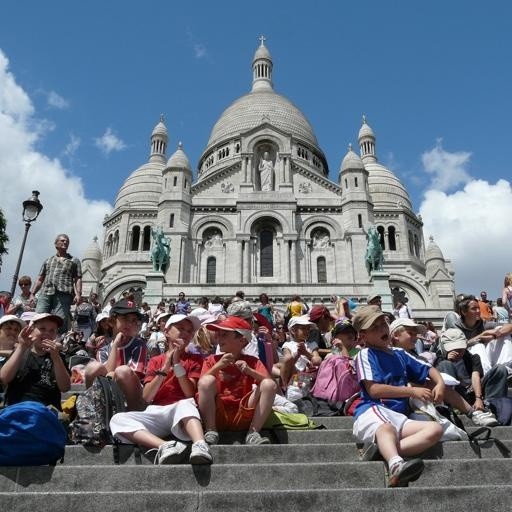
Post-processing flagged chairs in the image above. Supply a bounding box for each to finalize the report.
[202,430,224,446]
[473,409,497,426]
[189,440,214,466]
[386,455,426,487]
[244,428,272,446]
[146,440,188,464]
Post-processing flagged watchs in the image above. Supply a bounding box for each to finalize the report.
[476,394,484,400]
[155,368,167,376]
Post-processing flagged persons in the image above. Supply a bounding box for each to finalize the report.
[258,151,274,191]
[155,230,171,262]
[0,276,512,488]
[29,236,83,336]
[362,225,380,258]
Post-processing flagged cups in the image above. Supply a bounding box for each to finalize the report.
[179,294,185,297]
[57,240,69,243]
[18,282,30,287]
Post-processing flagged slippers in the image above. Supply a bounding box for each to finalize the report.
[74,375,124,446]
[0,400,68,467]
[312,353,359,403]
[76,301,96,323]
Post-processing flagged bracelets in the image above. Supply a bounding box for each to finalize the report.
[174,363,186,376]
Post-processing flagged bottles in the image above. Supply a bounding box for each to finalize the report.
[287,316,316,333]
[95,313,111,324]
[365,293,381,304]
[387,317,428,337]
[440,328,469,352]
[205,316,254,344]
[110,300,149,325]
[29,312,64,330]
[0,314,27,329]
[351,305,394,333]
[164,314,202,332]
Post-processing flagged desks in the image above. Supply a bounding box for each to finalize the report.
[8,188,44,300]
